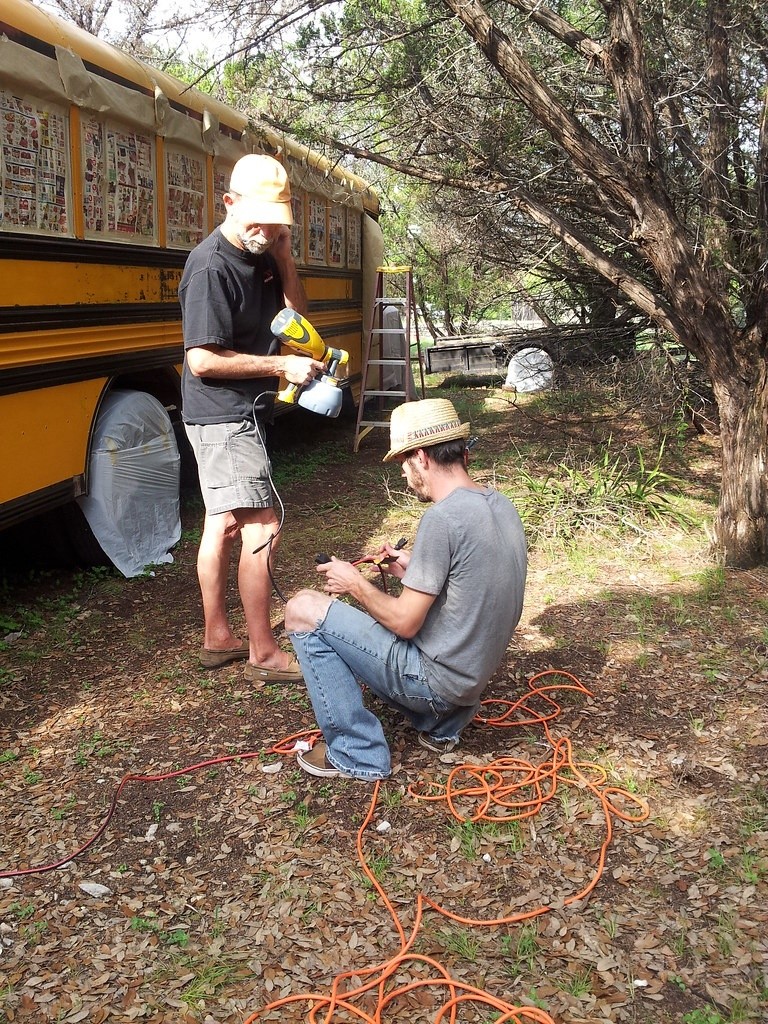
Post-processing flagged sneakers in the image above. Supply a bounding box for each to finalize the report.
[297,742,355,778]
[418,704,482,753]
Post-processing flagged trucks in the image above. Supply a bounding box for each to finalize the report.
[1,0,407,568]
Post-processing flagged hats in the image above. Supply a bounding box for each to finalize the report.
[230,154,294,224]
[382,399,471,462]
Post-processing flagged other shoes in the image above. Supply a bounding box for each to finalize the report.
[199,635,249,669]
[244,654,305,685]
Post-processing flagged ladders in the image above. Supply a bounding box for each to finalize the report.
[351,265,427,454]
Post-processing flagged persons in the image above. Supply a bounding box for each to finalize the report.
[180,153,349,679]
[285,399,527,782]
